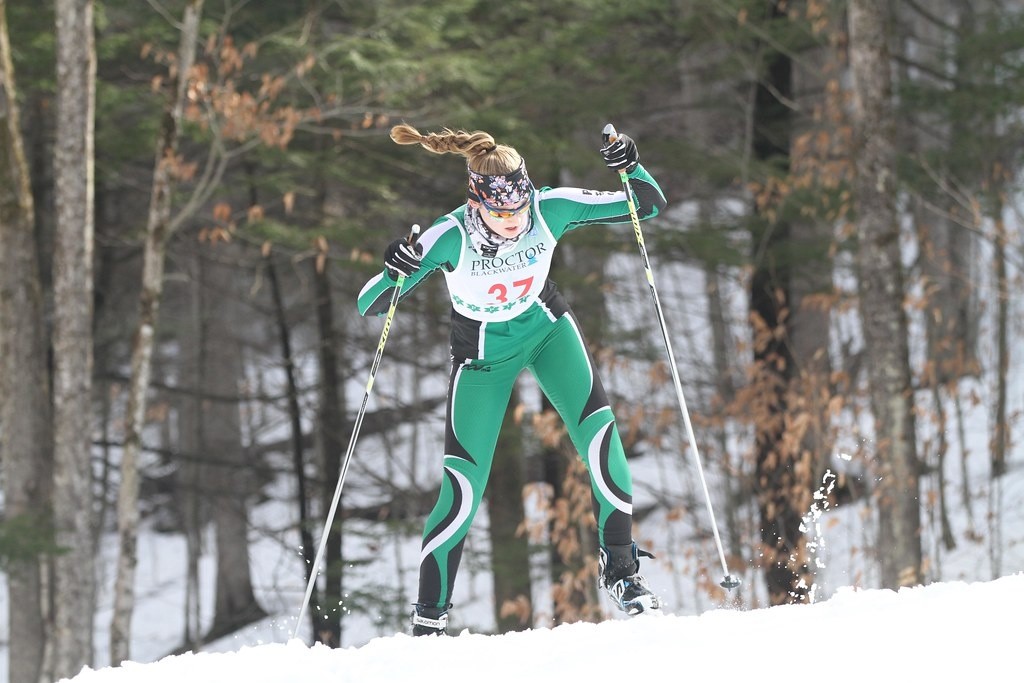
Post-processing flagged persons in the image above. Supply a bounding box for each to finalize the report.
[353,123,670,635]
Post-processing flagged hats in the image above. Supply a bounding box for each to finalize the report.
[465,157,532,207]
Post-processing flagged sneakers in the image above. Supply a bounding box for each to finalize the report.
[597,563,660,616]
[410,607,449,636]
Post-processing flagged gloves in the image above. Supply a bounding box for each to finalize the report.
[383,237,423,278]
[600,133,640,173]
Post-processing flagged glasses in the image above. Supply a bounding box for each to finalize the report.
[471,178,536,220]
